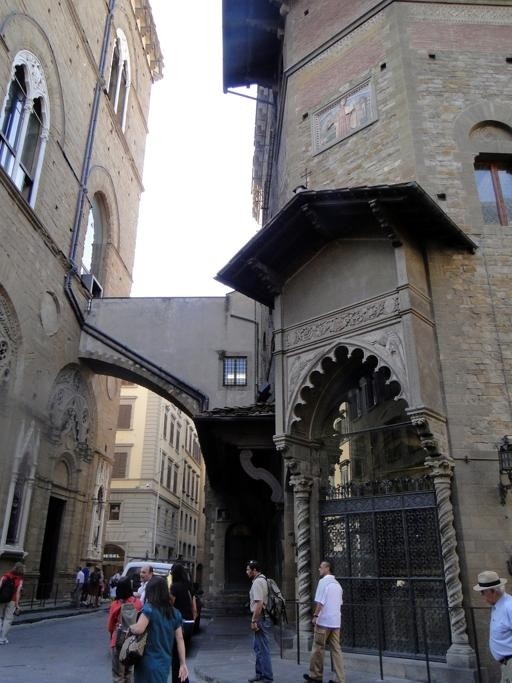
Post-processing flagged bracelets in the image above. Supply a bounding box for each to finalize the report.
[313,612,318,617]
[251,620,257,623]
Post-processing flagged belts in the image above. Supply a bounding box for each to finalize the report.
[500,656,512,664]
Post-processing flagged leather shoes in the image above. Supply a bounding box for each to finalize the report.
[248,677,256,682]
[253,679,271,683]
[303,673,322,683]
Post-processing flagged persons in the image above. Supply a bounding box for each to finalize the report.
[245,560,275,682]
[304,559,346,682]
[1,562,25,644]
[471,571,511,682]
[72,555,200,681]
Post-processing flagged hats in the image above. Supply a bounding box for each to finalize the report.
[472,570,508,592]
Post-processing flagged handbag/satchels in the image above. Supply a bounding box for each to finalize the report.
[119,630,148,664]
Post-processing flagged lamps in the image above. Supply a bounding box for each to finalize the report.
[498,435,512,505]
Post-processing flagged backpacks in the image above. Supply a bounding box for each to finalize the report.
[115,597,137,649]
[0,575,17,603]
[259,575,286,625]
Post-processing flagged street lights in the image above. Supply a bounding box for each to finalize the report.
[145,481,161,559]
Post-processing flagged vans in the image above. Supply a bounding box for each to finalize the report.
[110,560,202,632]
